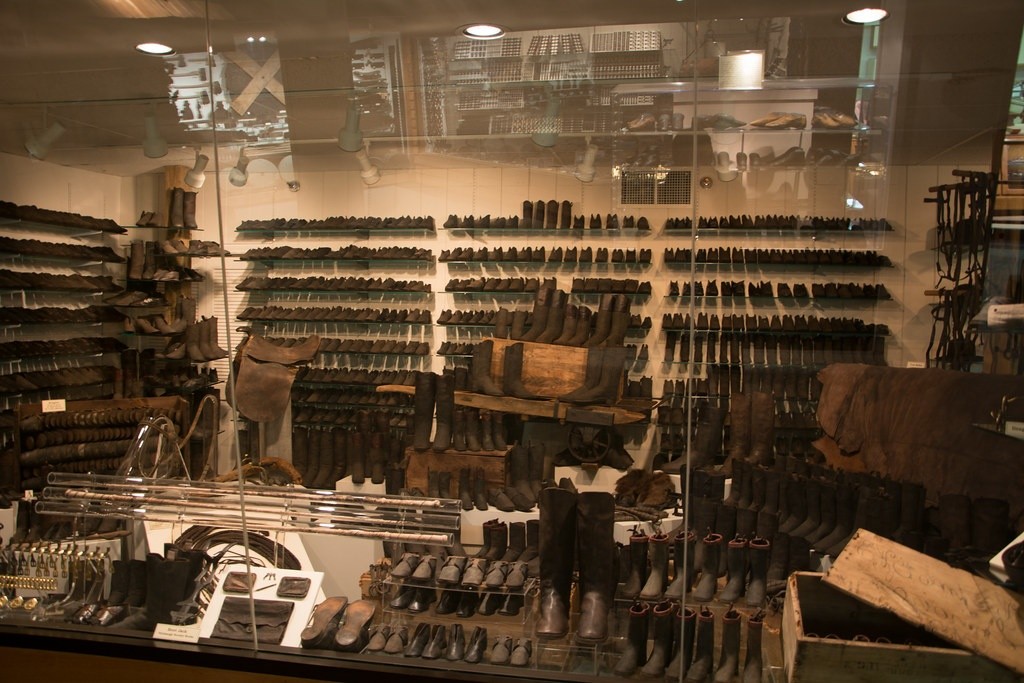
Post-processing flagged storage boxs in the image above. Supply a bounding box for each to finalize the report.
[779,527,1024,682]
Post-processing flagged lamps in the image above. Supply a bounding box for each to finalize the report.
[184,144,210,187]
[22,120,71,162]
[531,97,562,147]
[717,48,764,90]
[226,144,250,187]
[357,150,382,185]
[718,152,739,184]
[339,104,366,151]
[575,144,599,181]
[133,104,168,160]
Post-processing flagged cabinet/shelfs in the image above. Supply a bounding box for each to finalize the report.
[608,80,885,222]
[437,227,655,360]
[110,223,232,393]
[661,227,893,434]
[0,216,127,405]
[225,225,432,357]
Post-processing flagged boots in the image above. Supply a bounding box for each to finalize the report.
[452,287,652,512]
[535,477,577,638]
[640,601,674,678]
[714,601,741,683]
[613,601,650,675]
[575,492,615,645]
[742,608,768,683]
[686,604,714,683]
[664,607,697,681]
[624,332,1011,606]
[294,371,456,499]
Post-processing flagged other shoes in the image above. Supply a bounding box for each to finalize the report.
[625,111,866,168]
[236,215,433,428]
[0,187,226,631]
[662,215,892,335]
[437,200,652,360]
[300,518,541,667]
